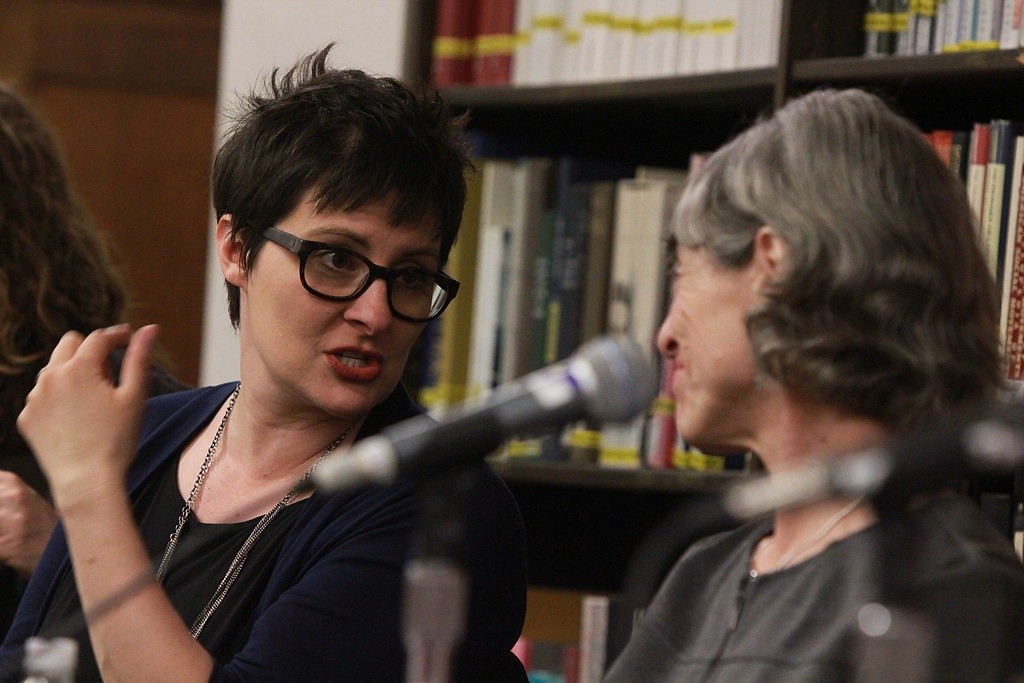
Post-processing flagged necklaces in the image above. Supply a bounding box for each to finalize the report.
[771,489,871,575]
[156,381,362,643]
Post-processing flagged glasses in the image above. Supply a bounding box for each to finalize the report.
[260,226,460,323]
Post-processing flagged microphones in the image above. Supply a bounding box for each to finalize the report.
[722,417,1024,522]
[304,334,666,497]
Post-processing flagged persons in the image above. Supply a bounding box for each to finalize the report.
[0,44,525,683]
[601,89,1024,683]
[0,86,190,645]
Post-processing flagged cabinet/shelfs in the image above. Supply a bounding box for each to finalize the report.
[399,0,1024,497]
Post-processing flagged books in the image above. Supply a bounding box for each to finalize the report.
[413,0,1024,683]
